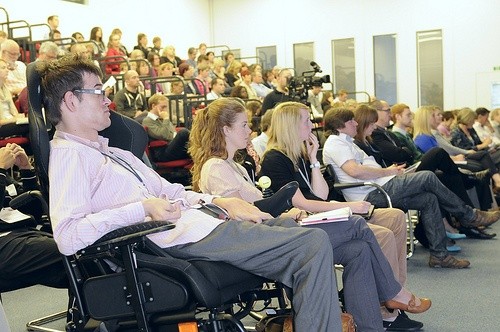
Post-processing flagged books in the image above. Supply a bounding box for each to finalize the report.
[299,199,376,228]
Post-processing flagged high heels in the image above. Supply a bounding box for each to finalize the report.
[493,187,500,198]
[384,292,432,313]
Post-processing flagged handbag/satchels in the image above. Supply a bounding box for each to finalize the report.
[283,313,356,332]
[413,215,429,245]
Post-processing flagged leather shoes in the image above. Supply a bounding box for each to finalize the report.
[459,227,492,238]
[464,175,480,189]
[446,244,461,252]
[445,230,466,239]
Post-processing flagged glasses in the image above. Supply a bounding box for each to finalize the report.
[5,50,21,58]
[64,87,106,102]
[380,108,390,112]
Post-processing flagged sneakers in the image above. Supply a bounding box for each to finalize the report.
[461,210,500,229]
[474,168,491,182]
[383,310,423,331]
[429,254,470,268]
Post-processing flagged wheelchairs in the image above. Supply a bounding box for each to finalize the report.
[9,190,292,332]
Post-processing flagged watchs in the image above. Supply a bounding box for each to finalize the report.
[309,161,321,169]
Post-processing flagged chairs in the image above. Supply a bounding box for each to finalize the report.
[326,163,416,268]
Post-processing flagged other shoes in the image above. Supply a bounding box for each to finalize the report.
[482,230,496,236]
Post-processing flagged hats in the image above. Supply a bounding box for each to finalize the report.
[476,108,491,114]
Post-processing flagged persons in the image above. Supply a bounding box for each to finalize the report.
[187,96,433,332]
[320,107,500,270]
[40,61,343,332]
[255,100,423,332]
[0,14,349,162]
[0,141,69,288]
[351,98,500,240]
